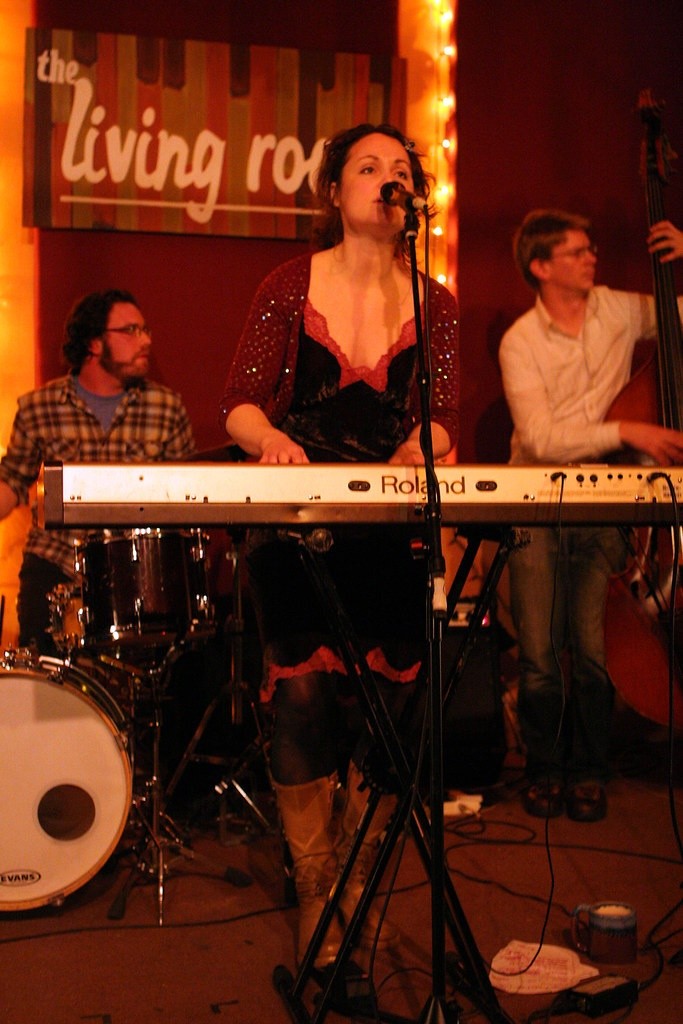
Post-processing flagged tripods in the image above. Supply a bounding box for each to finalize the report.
[104,554,278,925]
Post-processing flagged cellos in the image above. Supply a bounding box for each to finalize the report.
[596,83,683,731]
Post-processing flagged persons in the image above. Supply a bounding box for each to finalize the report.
[500,210,683,818]
[218,124,470,979]
[0,283,203,661]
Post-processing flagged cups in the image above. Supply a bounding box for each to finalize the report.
[573,902,638,965]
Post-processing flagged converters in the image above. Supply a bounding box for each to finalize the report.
[549,973,640,1018]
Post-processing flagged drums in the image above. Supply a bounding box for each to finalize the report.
[52,581,83,647]
[0,648,134,914]
[80,525,207,647]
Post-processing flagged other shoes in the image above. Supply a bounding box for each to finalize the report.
[565,770,605,821]
[522,761,566,817]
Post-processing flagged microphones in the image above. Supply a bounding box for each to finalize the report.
[274,526,335,553]
[378,181,431,212]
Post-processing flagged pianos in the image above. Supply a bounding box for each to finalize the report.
[40,457,682,1024]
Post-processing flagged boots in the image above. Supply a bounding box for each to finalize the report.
[268,762,346,968]
[339,757,402,949]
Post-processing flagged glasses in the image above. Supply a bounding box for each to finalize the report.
[103,323,151,338]
[542,245,597,259]
